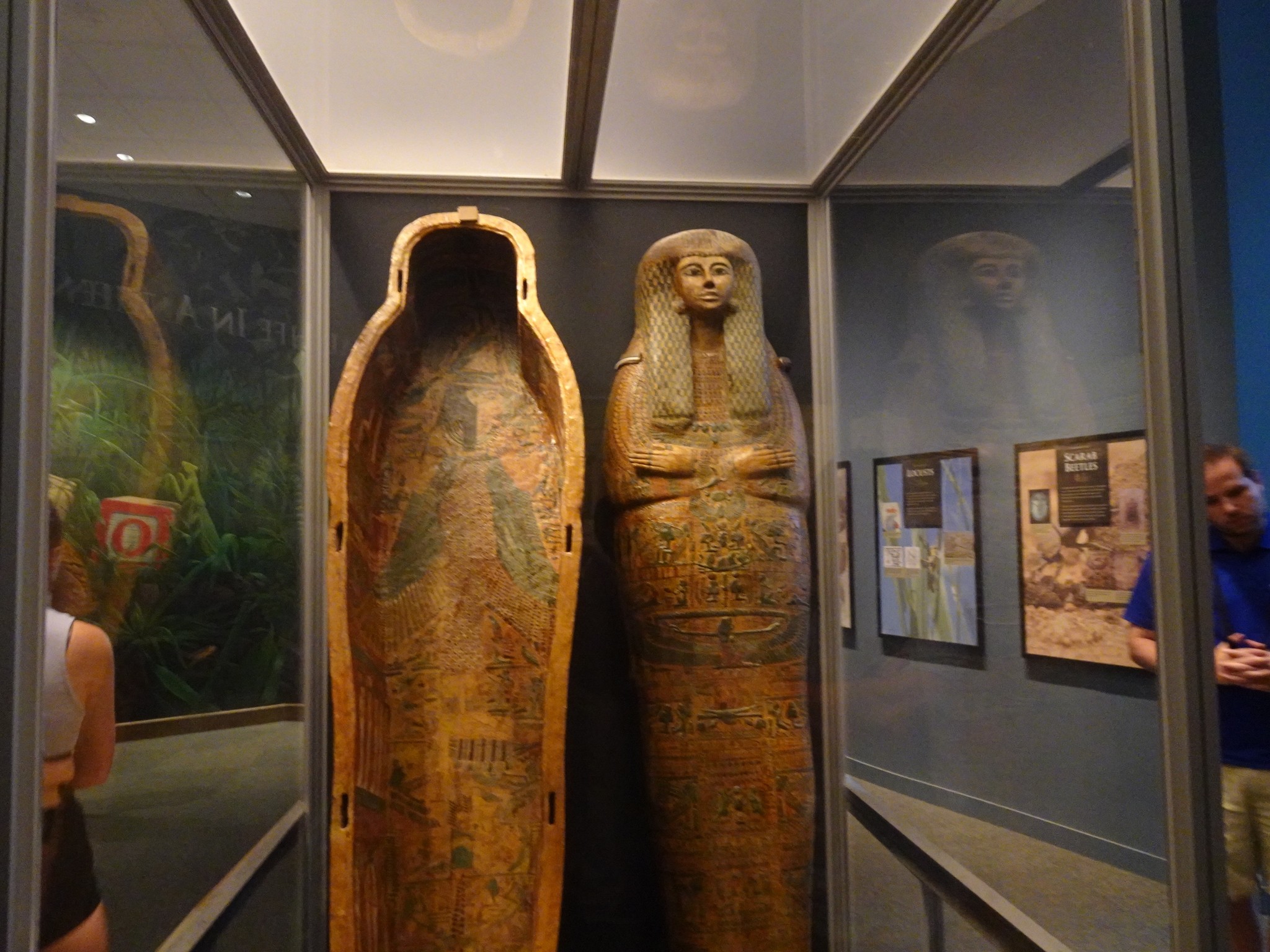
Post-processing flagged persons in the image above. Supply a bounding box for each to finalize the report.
[1120,444,1270,952]
[882,232,1100,839]
[605,228,817,946]
[38,497,115,952]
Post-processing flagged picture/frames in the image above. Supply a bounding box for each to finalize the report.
[875,449,983,654]
[1013,428,1156,679]
[837,458,857,636]
